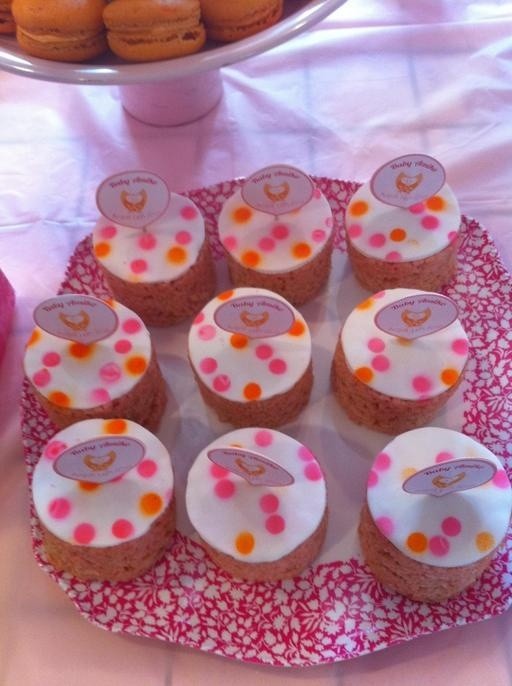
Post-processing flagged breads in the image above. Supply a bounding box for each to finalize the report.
[0,0,282,64]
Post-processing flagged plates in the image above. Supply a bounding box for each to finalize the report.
[20,175,511,669]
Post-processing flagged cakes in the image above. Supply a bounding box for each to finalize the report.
[184,428,328,583]
[91,170,219,328]
[356,427,512,603]
[22,295,168,435]
[218,165,335,307]
[345,152,464,295]
[331,288,473,437]
[32,415,176,583]
[188,287,313,427]
